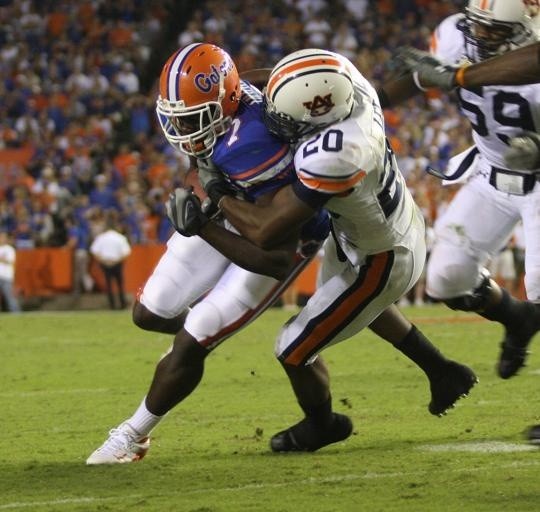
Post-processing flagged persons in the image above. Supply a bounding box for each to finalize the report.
[186,48,479,453]
[377,0,539,443]
[83,44,331,463]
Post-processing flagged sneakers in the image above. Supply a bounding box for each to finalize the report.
[86,419,150,465]
[429,365,479,418]
[499,303,540,378]
[527,424,540,445]
[270,412,353,452]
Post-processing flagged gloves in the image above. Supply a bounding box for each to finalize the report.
[395,42,469,93]
[197,158,234,209]
[165,184,212,237]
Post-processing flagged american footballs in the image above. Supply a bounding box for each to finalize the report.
[184,167,210,205]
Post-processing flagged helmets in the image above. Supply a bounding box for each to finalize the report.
[263,48,354,135]
[155,41,242,132]
[456,0,533,63]
